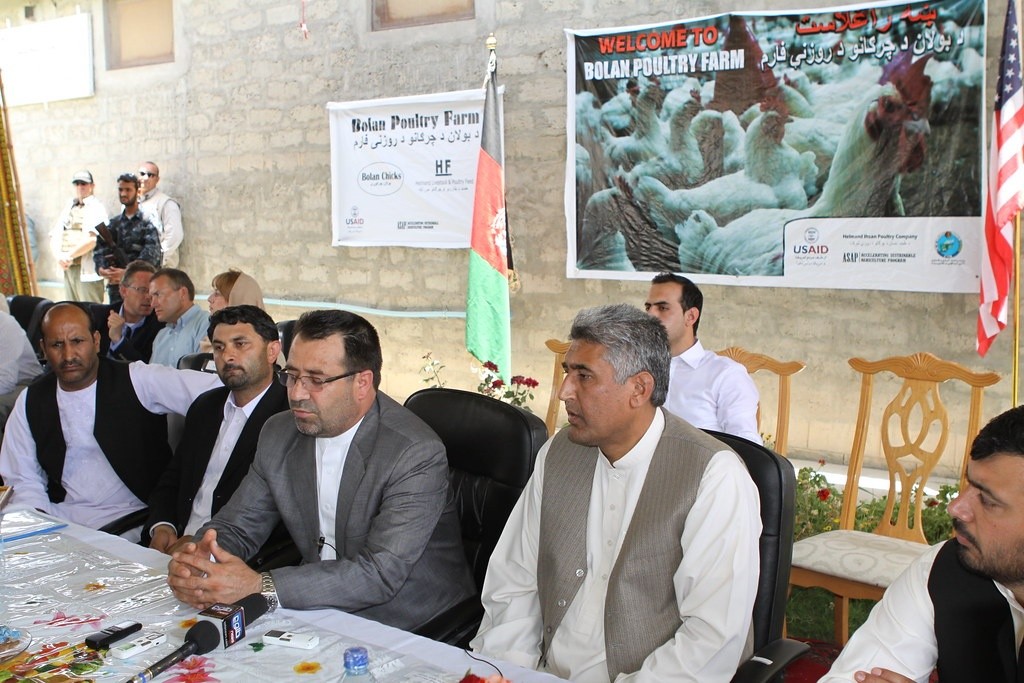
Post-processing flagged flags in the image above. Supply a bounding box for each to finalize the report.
[464,48,511,390]
[975,1,1024,357]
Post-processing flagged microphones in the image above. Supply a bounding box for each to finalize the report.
[125,593,270,683]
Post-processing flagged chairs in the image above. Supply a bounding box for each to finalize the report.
[403,388,548,640]
[714,347,806,456]
[781,353,1001,646]
[695,428,811,683]
[542,337,576,437]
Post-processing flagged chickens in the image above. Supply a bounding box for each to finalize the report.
[911,20,984,116]
[675,45,936,276]
[575,70,875,273]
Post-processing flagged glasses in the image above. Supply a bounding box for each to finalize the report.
[139,172,157,178]
[127,286,150,294]
[276,368,362,392]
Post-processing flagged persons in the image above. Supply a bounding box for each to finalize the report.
[90,260,267,368]
[0,301,225,546]
[468,301,763,683]
[135,161,184,270]
[644,269,763,446]
[137,301,292,555]
[0,308,45,487]
[49,166,110,304]
[165,309,478,641]
[815,404,1024,683]
[93,173,164,305]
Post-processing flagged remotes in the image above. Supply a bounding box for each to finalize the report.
[85,620,142,649]
[111,631,168,660]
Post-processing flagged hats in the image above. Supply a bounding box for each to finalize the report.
[71,170,93,183]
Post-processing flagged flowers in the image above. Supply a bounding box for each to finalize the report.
[793,458,962,647]
[417,347,538,412]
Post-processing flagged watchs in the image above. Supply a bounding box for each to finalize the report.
[259,572,278,613]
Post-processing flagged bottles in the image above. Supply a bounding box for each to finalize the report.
[336,646,380,683]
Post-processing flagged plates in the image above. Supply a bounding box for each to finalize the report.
[0,626,31,662]
